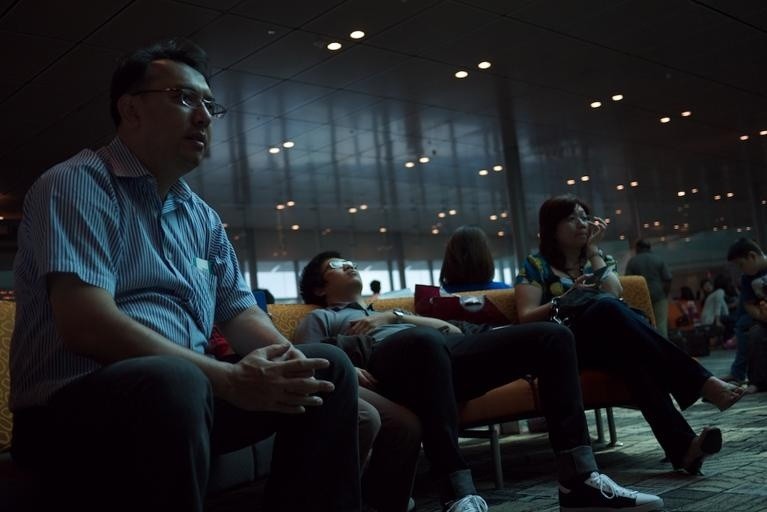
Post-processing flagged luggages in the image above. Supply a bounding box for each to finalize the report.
[676,327,710,355]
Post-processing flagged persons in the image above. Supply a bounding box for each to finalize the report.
[370,280,380,294]
[669,237,767,394]
[625,240,672,337]
[8,42,362,511]
[515,196,748,474]
[294,251,664,511]
[440,225,509,293]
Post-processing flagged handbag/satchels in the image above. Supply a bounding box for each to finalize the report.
[558,287,618,319]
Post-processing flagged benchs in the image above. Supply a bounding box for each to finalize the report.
[2,272,658,497]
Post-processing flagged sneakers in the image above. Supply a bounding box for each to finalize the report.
[451,495,481,512]
[559,474,664,511]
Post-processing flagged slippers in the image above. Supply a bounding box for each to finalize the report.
[702,383,747,411]
[686,428,722,474]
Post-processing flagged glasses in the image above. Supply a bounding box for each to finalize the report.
[130,88,227,119]
[322,261,358,278]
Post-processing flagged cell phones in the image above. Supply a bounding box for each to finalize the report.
[585,267,608,285]
